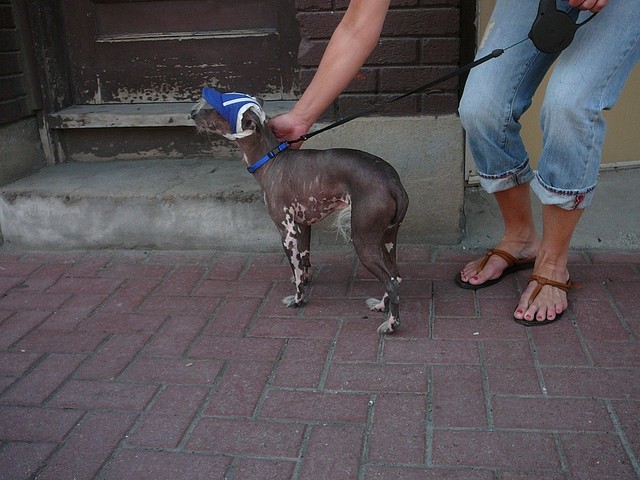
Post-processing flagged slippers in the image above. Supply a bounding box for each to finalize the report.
[456,248,537,290]
[513,271,572,327]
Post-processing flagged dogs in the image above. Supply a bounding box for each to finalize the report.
[188,91,410,335]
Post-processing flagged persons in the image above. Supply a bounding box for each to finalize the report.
[266,0,640,328]
